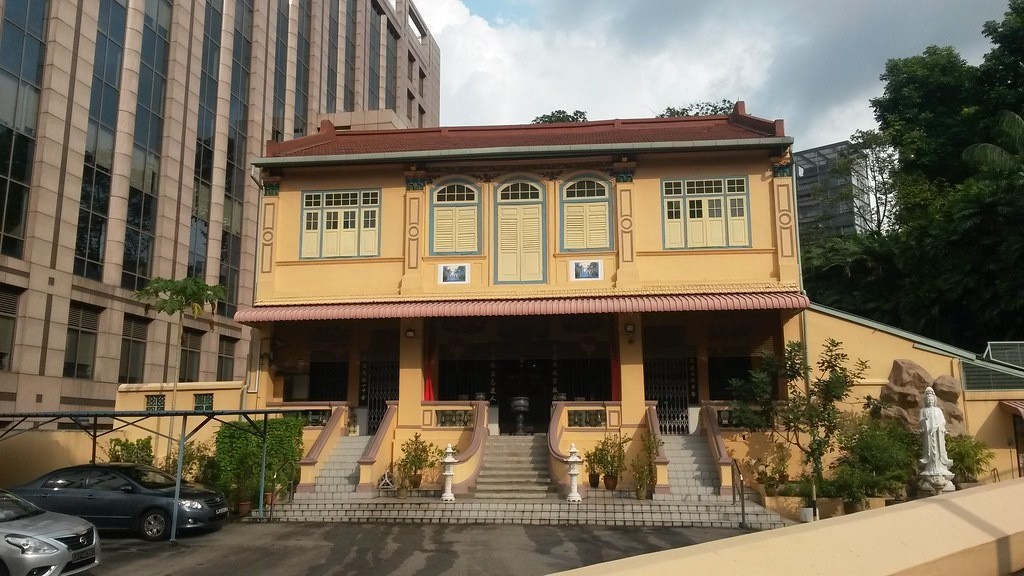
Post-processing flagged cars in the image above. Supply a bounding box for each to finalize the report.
[0,487,102,576]
[8,463,229,540]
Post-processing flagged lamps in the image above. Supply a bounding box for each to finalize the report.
[624,324,635,333]
[405,328,416,338]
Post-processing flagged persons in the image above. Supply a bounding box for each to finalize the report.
[916,387,954,473]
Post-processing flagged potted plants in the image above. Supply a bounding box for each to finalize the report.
[394,458,418,499]
[234,480,261,516]
[401,432,438,489]
[278,471,294,500]
[744,456,771,484]
[631,453,652,500]
[828,427,915,515]
[946,439,996,490]
[264,484,279,506]
[594,431,634,491]
[583,449,603,488]
[764,476,779,497]
[771,441,790,484]
